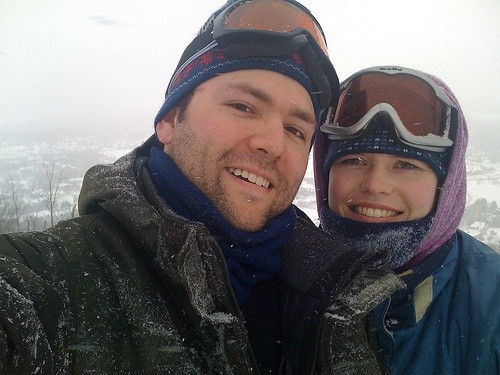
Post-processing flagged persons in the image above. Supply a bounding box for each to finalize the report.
[1,0,407,375]
[313,64,500,375]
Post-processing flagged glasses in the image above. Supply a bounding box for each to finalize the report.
[320,65,453,154]
[163,0,340,112]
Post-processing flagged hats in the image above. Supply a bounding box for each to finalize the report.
[153,0,320,152]
[324,115,448,182]
